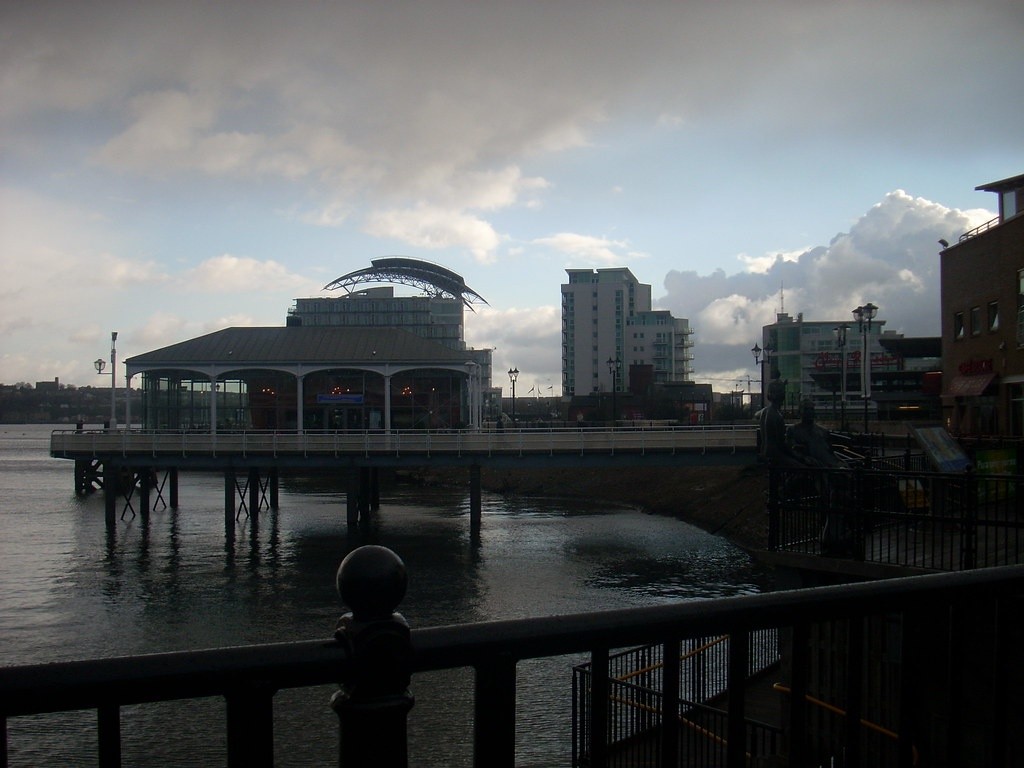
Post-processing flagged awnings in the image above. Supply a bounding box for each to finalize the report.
[939,373,997,397]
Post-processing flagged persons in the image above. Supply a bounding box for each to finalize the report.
[754,380,854,543]
[496,417,586,433]
[215,418,232,433]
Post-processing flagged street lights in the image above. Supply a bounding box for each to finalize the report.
[751,341,776,412]
[832,323,852,434]
[851,302,879,435]
[606,357,623,427]
[507,367,519,423]
[95,331,118,433]
